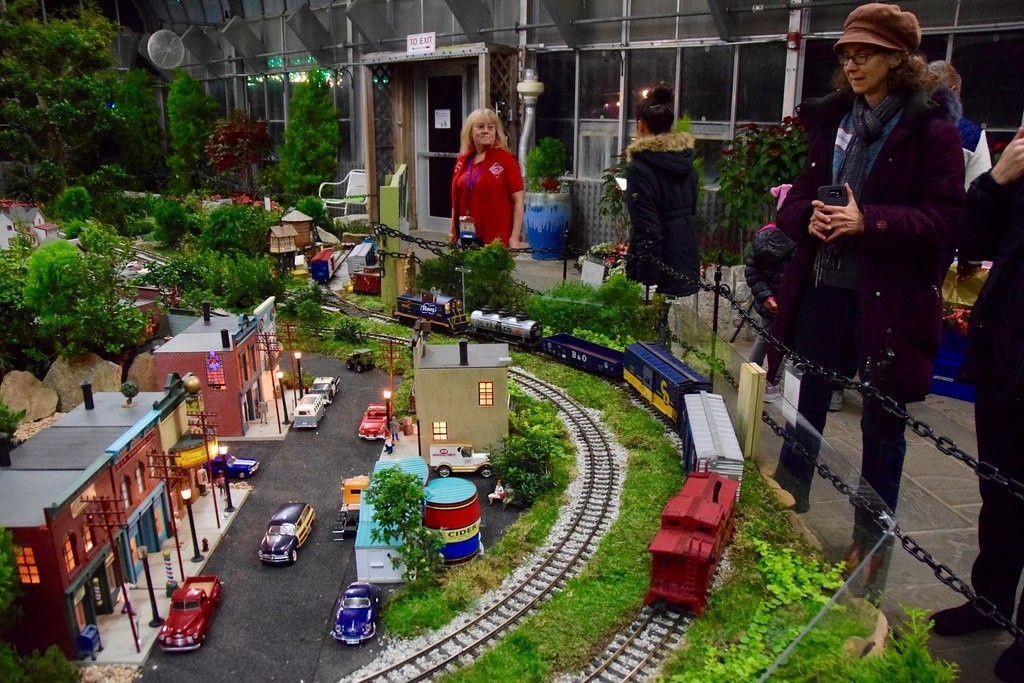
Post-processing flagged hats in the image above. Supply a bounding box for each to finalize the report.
[770,183,793,212]
[833,3,921,56]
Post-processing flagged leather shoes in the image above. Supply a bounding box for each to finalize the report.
[842,540,886,596]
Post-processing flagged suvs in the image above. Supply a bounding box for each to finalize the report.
[345,349,375,373]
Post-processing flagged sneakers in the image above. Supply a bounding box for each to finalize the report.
[828,389,848,411]
[764,382,783,402]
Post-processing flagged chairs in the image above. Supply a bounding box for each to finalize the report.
[319,169,366,218]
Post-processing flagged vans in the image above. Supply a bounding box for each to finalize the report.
[428,444,492,478]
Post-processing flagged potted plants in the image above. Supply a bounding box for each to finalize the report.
[524,138,576,262]
[411,415,417,434]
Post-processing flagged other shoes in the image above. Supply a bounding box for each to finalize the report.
[929,601,1012,636]
[996,639,1024,672]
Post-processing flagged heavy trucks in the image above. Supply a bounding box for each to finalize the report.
[347,243,376,277]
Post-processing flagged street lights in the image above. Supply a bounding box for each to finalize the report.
[180,485,205,562]
[275,370,290,424]
[294,350,303,400]
[137,546,166,627]
[219,443,236,512]
[384,389,392,433]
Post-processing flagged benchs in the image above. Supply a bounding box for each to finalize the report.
[487,486,526,510]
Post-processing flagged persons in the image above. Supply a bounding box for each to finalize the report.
[216,470,227,496]
[501,483,516,512]
[381,426,394,453]
[448,108,531,256]
[259,401,269,424]
[744,222,846,411]
[115,577,139,616]
[766,3,963,596]
[390,417,401,441]
[927,127,1024,678]
[488,480,504,505]
[613,82,702,351]
[929,59,992,194]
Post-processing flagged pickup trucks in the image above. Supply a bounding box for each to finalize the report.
[156,574,225,651]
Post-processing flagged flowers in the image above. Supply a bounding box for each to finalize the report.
[569,154,631,274]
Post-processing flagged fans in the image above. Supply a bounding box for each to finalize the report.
[147,29,185,70]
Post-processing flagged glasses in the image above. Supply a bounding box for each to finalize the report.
[838,48,884,66]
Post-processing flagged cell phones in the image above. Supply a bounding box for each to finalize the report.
[614,177,627,191]
[817,185,848,237]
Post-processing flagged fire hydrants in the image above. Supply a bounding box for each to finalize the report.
[385,428,390,439]
[304,384,308,393]
[202,538,209,552]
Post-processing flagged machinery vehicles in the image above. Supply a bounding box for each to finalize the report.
[332,475,371,542]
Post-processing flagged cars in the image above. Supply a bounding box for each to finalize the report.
[293,375,341,427]
[257,501,316,562]
[329,581,380,644]
[358,404,391,440]
[203,453,260,479]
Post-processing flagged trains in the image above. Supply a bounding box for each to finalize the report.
[392,287,745,619]
[310,250,336,283]
